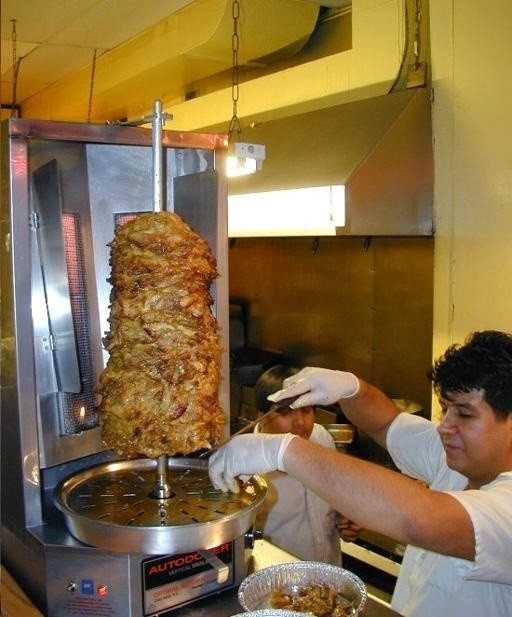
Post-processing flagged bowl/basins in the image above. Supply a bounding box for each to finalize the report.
[390,397,426,416]
[239,559,367,617]
[229,609,316,616]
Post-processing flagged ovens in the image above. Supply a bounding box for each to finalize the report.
[1,120,267,616]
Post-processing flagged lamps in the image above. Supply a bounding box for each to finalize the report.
[217,0,269,180]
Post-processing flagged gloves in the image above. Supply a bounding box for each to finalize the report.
[206,432,298,495]
[266,365,360,410]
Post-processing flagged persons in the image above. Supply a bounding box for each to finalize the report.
[243,362,369,579]
[203,327,511,615]
